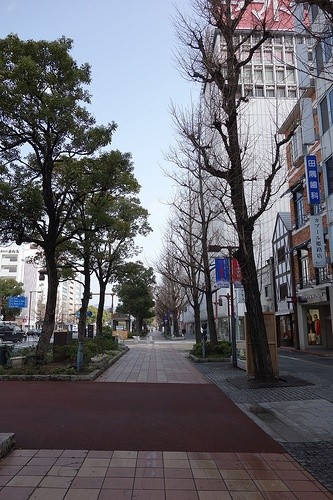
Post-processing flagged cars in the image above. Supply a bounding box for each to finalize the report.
[27,329,38,336]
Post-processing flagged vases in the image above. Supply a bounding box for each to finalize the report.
[10,356,26,369]
[231,356,247,370]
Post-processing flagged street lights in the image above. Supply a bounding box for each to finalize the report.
[206,244,240,368]
[28,290,41,330]
[89,291,116,333]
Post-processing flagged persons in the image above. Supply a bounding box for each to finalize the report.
[201,326,204,336]
[313,314,321,345]
[12,325,17,341]
[307,314,317,345]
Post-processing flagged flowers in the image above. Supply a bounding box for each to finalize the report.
[239,349,246,360]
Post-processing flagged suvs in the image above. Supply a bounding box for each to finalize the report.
[0,321,27,344]
